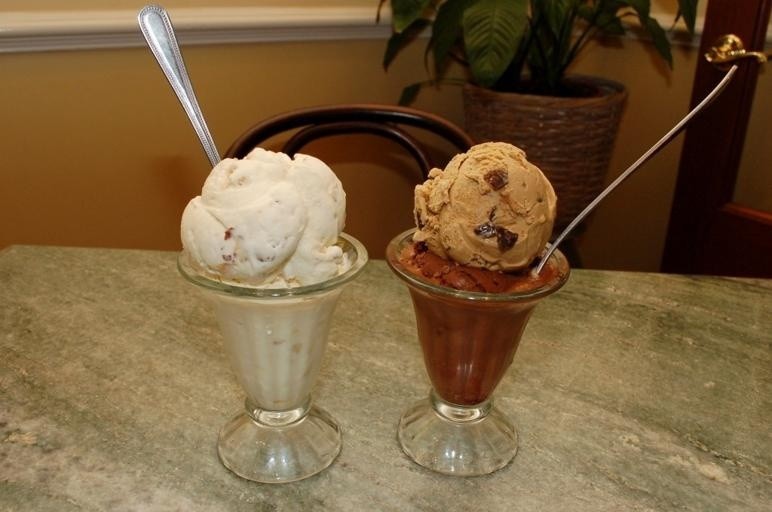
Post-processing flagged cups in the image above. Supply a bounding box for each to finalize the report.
[382,227,570,478]
[179,230,371,487]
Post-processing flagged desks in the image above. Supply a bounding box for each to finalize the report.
[1,244,770,512]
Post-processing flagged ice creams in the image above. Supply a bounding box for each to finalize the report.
[400,141,558,405]
[180,145,348,410]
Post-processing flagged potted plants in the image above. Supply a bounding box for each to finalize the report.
[373,0,699,225]
